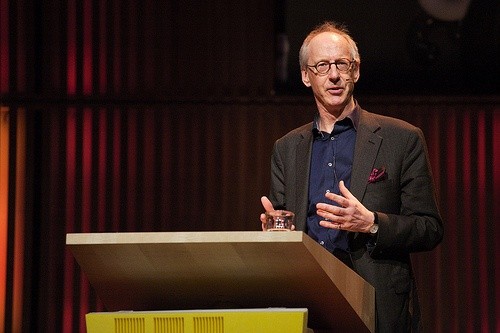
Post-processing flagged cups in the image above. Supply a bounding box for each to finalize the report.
[264,211,294,231]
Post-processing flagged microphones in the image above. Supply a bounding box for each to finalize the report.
[346,78,357,81]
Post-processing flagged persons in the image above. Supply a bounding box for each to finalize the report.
[260,22,443,332]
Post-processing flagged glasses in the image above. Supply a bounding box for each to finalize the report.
[305,58,356,74]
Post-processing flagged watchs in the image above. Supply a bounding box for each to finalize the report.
[368,211,380,237]
